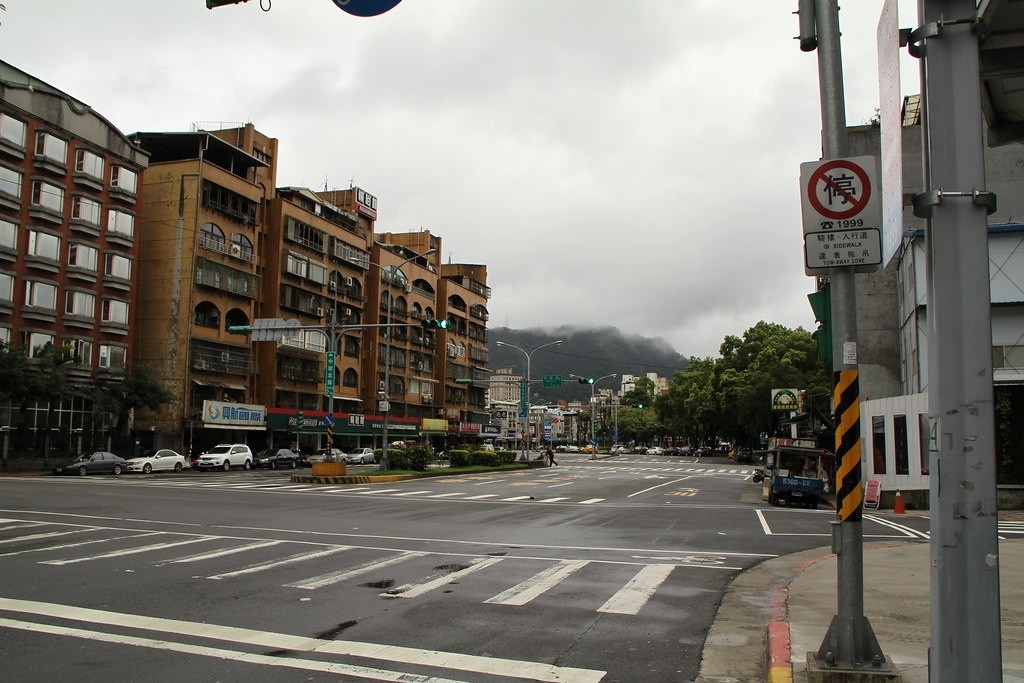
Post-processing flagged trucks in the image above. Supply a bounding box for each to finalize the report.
[753,437,835,509]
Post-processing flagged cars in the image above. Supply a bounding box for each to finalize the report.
[728,447,752,462]
[53,451,128,477]
[304,447,348,466]
[556,444,579,453]
[437,445,505,460]
[611,444,713,458]
[252,449,299,470]
[346,448,375,464]
[580,445,598,454]
[124,449,189,474]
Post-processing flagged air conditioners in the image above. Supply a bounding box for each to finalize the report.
[317,308,323,316]
[329,308,334,315]
[404,284,411,293]
[222,352,229,362]
[194,360,205,369]
[345,277,352,286]
[229,244,241,258]
[330,281,335,290]
[345,308,351,316]
[450,348,456,357]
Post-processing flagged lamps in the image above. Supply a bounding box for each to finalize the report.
[134,132,141,147]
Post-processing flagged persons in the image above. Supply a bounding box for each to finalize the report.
[545,446,559,467]
[533,451,544,460]
[732,448,737,462]
[804,460,823,478]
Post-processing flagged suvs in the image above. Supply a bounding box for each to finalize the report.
[195,444,253,471]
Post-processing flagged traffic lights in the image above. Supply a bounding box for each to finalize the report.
[578,378,594,384]
[632,404,646,409]
[422,318,451,330]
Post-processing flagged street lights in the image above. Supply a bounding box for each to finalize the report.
[496,341,562,460]
[570,373,618,458]
[347,249,436,458]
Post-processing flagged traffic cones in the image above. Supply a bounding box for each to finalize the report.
[892,489,907,514]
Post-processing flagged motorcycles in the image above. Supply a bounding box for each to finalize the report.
[753,468,764,483]
